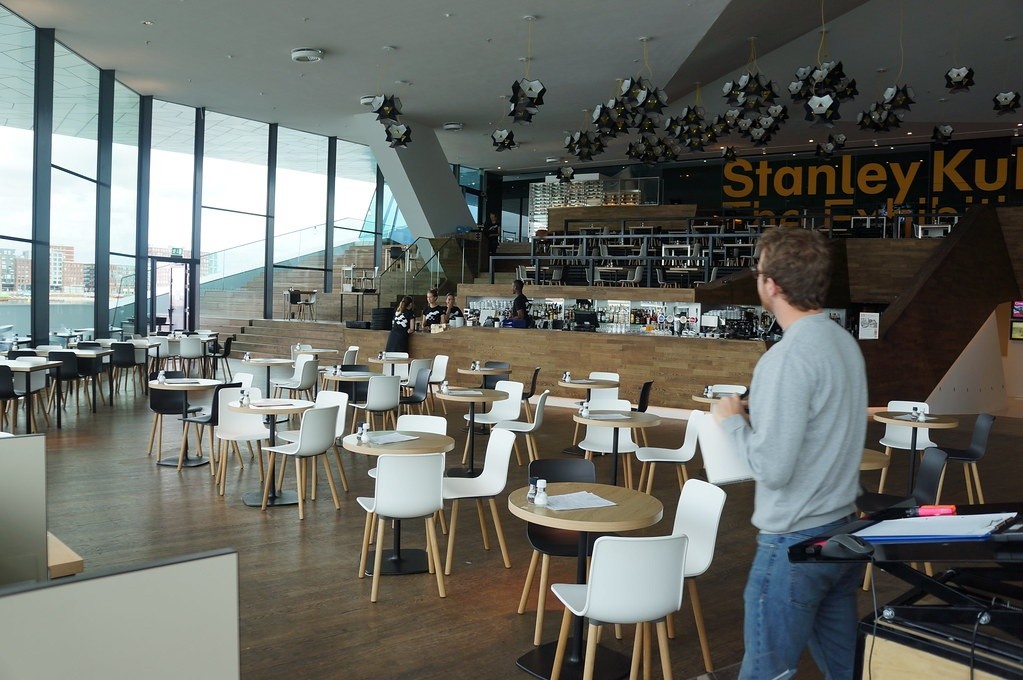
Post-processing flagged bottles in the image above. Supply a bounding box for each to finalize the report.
[470,360,480,371]
[239,389,251,407]
[331,364,342,377]
[157,366,167,384]
[578,400,589,419]
[469,299,841,341]
[703,384,716,400]
[297,343,301,351]
[378,351,387,361]
[913,407,926,422]
[355,421,371,444]
[440,380,449,395]
[561,371,572,383]
[243,351,251,361]
[527,476,549,508]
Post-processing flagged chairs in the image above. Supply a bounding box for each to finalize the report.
[288,290,302,321]
[389,246,406,273]
[408,245,421,272]
[0,317,996,680]
[297,289,317,321]
[514,218,958,289]
[340,267,379,292]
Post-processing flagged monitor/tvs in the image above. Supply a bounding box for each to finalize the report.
[573,310,600,329]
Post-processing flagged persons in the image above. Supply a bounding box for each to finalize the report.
[509,280,529,320]
[386,296,414,353]
[442,293,465,323]
[484,211,501,272]
[713,227,869,680]
[422,288,445,328]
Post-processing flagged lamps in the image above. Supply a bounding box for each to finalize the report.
[290,0,1023,185]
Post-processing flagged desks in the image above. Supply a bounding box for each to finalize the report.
[917,224,952,241]
[282,290,317,321]
[174,332,219,369]
[557,379,618,457]
[607,244,634,264]
[69,343,161,394]
[120,320,173,339]
[874,411,959,498]
[525,266,549,285]
[503,483,664,680]
[76,328,124,342]
[669,267,698,289]
[339,292,380,323]
[294,349,339,404]
[579,227,604,250]
[1,337,31,352]
[723,242,754,267]
[661,243,691,267]
[817,229,849,242]
[455,366,512,436]
[367,358,413,418]
[147,379,221,467]
[243,398,314,507]
[628,226,655,248]
[324,374,370,447]
[344,267,375,292]
[693,225,720,247]
[56,334,80,349]
[434,386,509,478]
[0,362,63,433]
[241,357,295,425]
[342,431,455,578]
[166,337,217,379]
[572,411,660,487]
[549,243,573,266]
[598,266,622,287]
[747,224,775,246]
[22,349,114,413]
[385,248,407,272]
[691,394,750,478]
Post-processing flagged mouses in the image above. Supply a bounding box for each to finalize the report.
[821,533,875,559]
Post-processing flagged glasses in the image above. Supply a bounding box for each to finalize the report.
[749,265,777,284]
